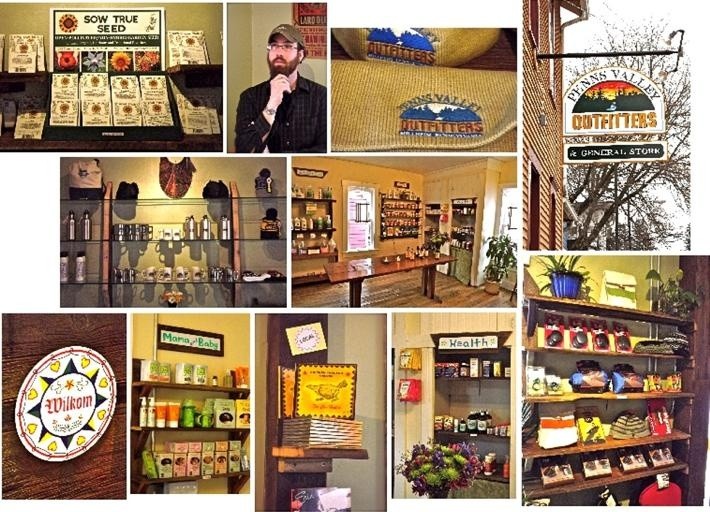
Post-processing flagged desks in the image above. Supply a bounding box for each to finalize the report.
[323,251,458,308]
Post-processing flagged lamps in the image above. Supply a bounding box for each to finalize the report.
[537,30,685,78]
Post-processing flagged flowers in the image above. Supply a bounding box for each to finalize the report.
[60,51,161,72]
[394,435,484,498]
[431,233,449,250]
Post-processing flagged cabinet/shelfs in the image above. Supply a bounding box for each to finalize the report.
[1,68,224,154]
[450,199,477,284]
[292,196,339,285]
[132,380,250,494]
[434,350,511,484]
[524,291,693,502]
[108,182,235,306]
[380,193,421,241]
[235,180,286,306]
[60,183,107,307]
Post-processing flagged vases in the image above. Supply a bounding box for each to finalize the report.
[435,248,440,260]
[425,485,451,498]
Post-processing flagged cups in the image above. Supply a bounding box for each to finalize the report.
[140,267,205,281]
[173,228,184,241]
[236,367,249,387]
[197,407,212,429]
[450,238,472,250]
[166,402,180,428]
[115,268,136,281]
[113,223,154,240]
[163,227,172,240]
[158,229,164,239]
[154,401,167,427]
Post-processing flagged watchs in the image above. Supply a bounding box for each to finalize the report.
[264,106,278,117]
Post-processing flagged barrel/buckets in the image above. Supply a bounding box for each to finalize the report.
[295,216,307,231]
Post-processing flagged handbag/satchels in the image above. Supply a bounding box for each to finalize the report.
[599,269,637,309]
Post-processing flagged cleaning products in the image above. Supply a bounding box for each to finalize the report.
[502,454,509,479]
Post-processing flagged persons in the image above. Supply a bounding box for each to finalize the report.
[235,23,327,153]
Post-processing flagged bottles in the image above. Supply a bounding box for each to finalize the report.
[457,207,475,215]
[223,368,233,387]
[295,214,331,230]
[74,250,87,283]
[459,418,467,432]
[67,209,77,240]
[291,184,332,199]
[502,454,509,479]
[467,409,493,433]
[387,187,420,201]
[405,243,429,260]
[495,424,510,436]
[484,452,497,476]
[213,375,218,386]
[80,209,93,241]
[60,251,69,283]
[184,214,231,240]
[453,226,472,236]
[435,245,441,259]
[182,398,196,428]
[461,362,469,376]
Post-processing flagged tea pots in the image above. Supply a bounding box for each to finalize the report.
[206,264,224,279]
[224,266,234,279]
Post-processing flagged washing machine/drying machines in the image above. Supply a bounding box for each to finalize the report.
[325,215,332,229]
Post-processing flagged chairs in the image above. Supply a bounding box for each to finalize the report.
[640,480,682,506]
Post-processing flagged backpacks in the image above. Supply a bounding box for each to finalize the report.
[68,159,106,199]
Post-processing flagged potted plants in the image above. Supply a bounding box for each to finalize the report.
[484,234,517,295]
[646,267,686,311]
[536,255,597,299]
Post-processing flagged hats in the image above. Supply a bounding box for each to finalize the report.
[268,24,304,47]
